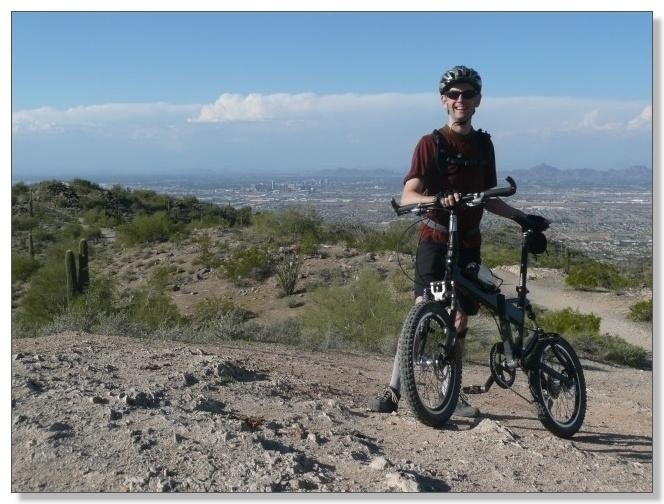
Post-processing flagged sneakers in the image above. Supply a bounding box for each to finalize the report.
[438,392,479,418]
[367,386,401,413]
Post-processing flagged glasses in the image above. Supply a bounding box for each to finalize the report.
[444,89,476,100]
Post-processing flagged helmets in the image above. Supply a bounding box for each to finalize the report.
[439,66,481,95]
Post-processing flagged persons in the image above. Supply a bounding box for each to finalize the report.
[367,65,552,418]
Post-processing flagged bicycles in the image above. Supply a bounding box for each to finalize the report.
[388,174,587,437]
